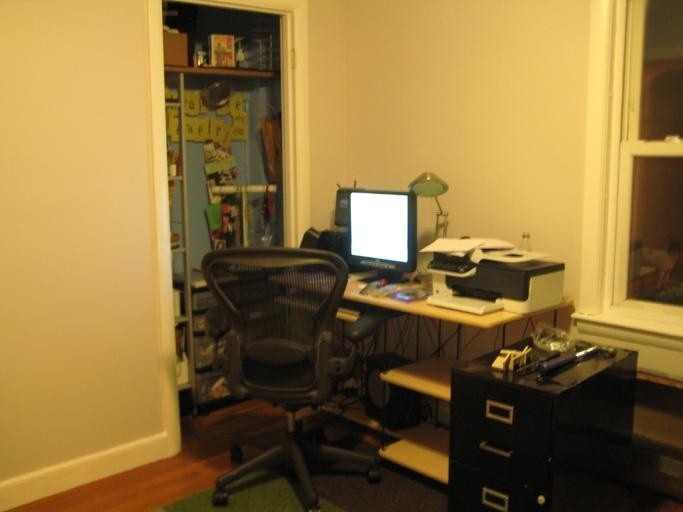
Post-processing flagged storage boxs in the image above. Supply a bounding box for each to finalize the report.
[164,30,188,69]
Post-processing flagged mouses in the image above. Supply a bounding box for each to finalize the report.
[360,278,396,296]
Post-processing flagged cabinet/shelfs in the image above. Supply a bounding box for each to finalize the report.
[163,68,280,419]
[632,368,683,456]
[447,335,637,511]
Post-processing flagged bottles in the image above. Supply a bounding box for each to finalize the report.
[521,231,530,253]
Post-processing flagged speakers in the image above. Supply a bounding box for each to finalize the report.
[365,353,420,431]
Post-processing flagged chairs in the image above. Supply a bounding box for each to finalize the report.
[201,247,382,512]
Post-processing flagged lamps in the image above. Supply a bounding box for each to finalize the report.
[409,170,449,240]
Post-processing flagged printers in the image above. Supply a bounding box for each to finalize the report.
[427,254,565,315]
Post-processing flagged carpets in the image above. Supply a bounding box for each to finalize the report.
[162,436,447,512]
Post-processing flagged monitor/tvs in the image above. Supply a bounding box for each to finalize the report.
[347,189,416,284]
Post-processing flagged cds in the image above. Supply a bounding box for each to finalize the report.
[396,288,426,301]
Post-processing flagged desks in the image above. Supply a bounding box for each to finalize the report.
[264,266,572,489]
